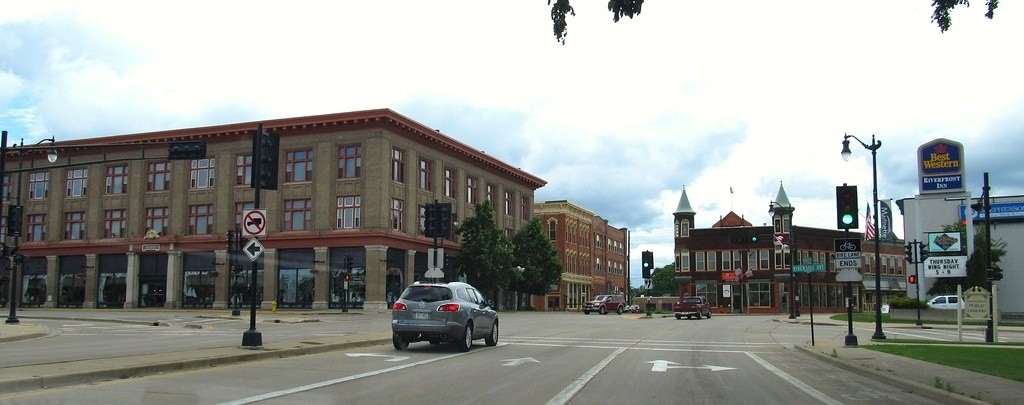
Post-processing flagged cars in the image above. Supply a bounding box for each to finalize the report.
[927,295,965,310]
[624,304,640,314]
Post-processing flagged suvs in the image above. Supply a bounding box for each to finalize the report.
[582,294,627,315]
[391,279,499,353]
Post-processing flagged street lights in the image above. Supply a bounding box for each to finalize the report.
[5,134,59,323]
[768,201,797,319]
[840,132,887,340]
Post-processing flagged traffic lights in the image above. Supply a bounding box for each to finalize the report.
[904,242,913,263]
[346,256,354,272]
[971,202,983,212]
[919,243,928,262]
[344,273,351,281]
[909,275,916,284]
[730,233,760,246]
[836,183,859,229]
[642,251,654,279]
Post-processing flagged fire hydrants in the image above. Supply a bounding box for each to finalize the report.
[271,300,278,312]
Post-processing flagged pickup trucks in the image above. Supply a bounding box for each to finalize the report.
[673,295,713,320]
